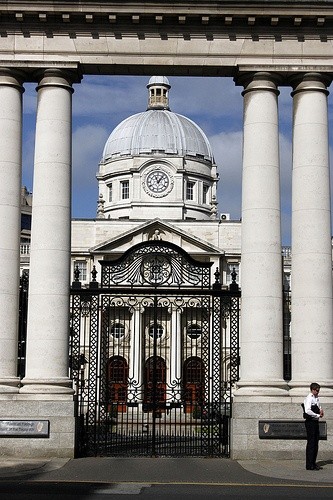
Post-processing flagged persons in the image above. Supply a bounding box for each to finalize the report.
[304,383,326,471]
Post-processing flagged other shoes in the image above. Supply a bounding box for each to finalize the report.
[306,467,319,470]
[316,467,323,469]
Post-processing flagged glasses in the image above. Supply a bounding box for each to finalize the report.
[313,389,319,392]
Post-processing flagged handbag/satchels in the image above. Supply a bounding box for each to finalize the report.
[300,403,320,421]
